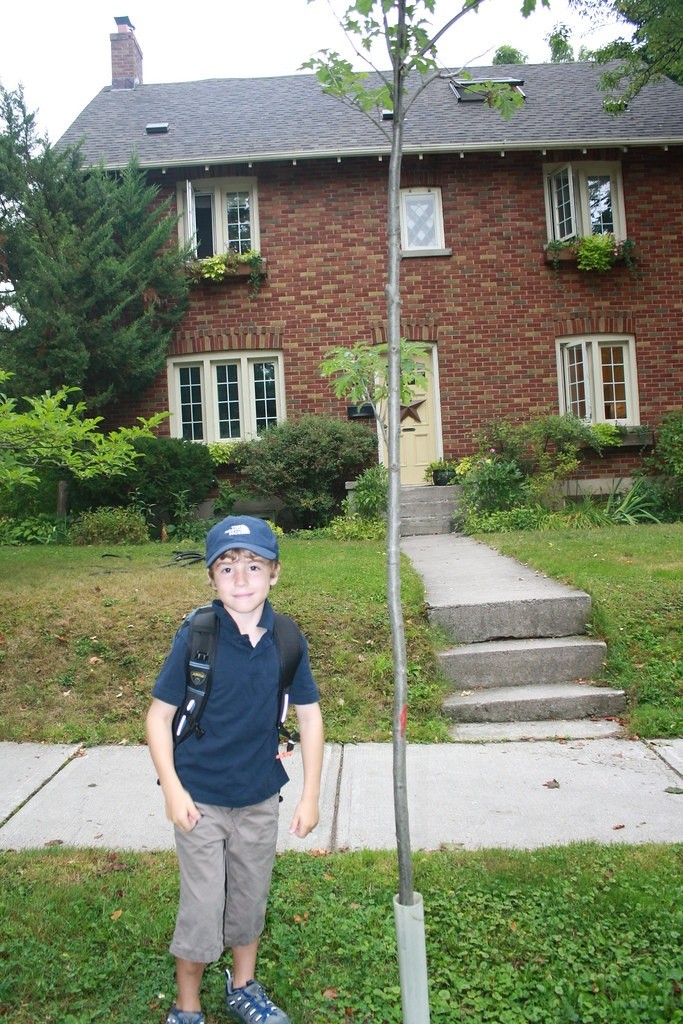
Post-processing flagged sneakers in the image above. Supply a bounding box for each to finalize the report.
[225,968,291,1024]
[164,1005,205,1024]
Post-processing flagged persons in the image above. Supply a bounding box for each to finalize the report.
[144,515,326,1024]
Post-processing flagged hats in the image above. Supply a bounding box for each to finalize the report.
[205,515,279,568]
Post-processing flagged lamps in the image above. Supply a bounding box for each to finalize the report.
[347,404,377,418]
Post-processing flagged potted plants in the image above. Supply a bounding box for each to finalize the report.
[423,459,467,487]
